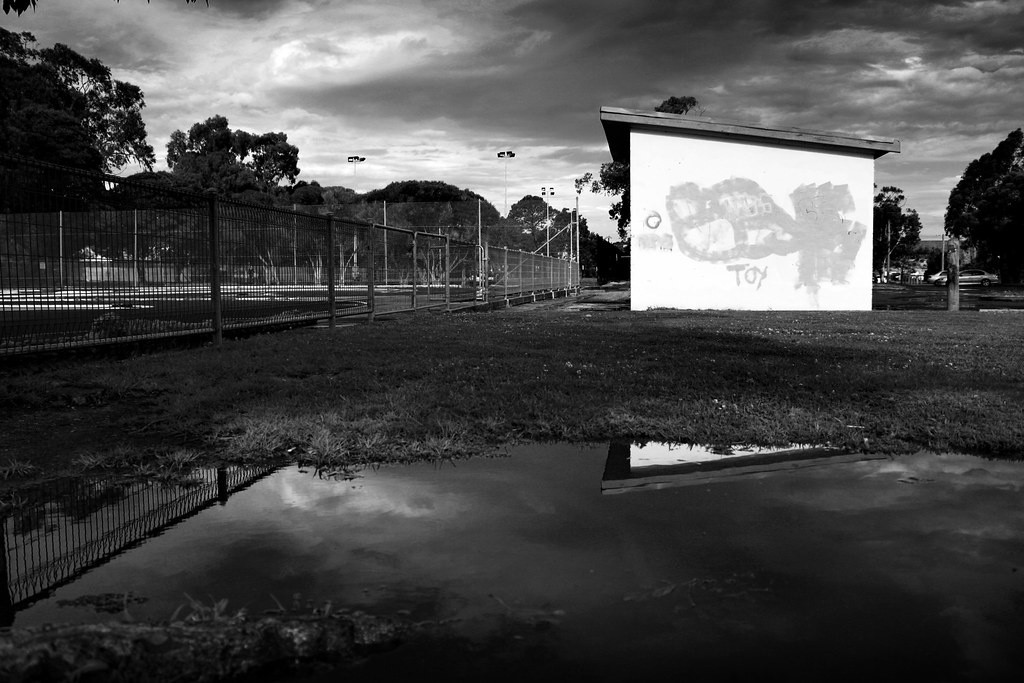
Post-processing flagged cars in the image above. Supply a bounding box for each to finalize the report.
[937,269,1001,289]
[926,269,948,286]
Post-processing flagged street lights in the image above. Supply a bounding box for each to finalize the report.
[496,150,516,249]
[540,185,555,257]
[347,155,366,224]
